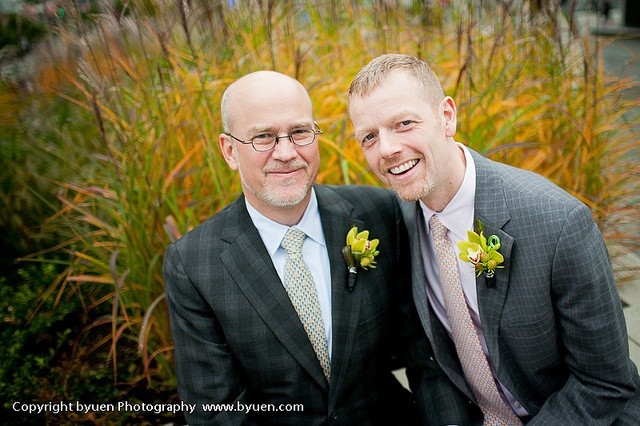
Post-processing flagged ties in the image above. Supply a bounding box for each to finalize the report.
[429,216,524,426]
[281,229,332,386]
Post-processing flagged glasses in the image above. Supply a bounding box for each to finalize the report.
[224,122,323,152]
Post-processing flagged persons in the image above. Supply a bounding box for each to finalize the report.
[163,70,483,426]
[346,54,640,426]
